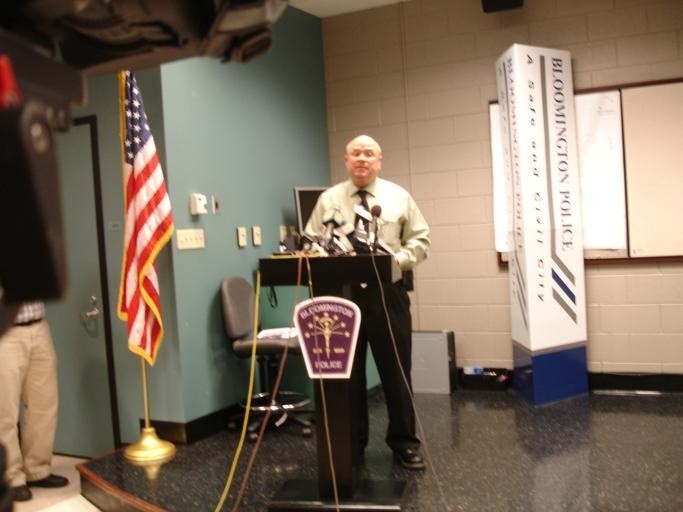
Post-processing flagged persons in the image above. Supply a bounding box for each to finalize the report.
[302,132,432,472]
[0,302,71,500]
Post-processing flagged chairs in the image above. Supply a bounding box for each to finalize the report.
[217,274,316,445]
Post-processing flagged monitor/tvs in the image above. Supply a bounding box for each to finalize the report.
[293,182,332,236]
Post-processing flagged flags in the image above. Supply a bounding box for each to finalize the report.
[115,70,175,367]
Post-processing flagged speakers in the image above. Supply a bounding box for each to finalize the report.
[480,0,524,13]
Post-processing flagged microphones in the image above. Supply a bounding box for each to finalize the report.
[316,205,390,255]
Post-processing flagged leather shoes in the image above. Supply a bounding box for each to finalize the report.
[390,440,427,471]
[8,471,68,502]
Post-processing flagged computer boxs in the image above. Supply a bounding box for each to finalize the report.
[407,328,457,396]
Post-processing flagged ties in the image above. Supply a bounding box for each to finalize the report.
[355,188,374,229]
